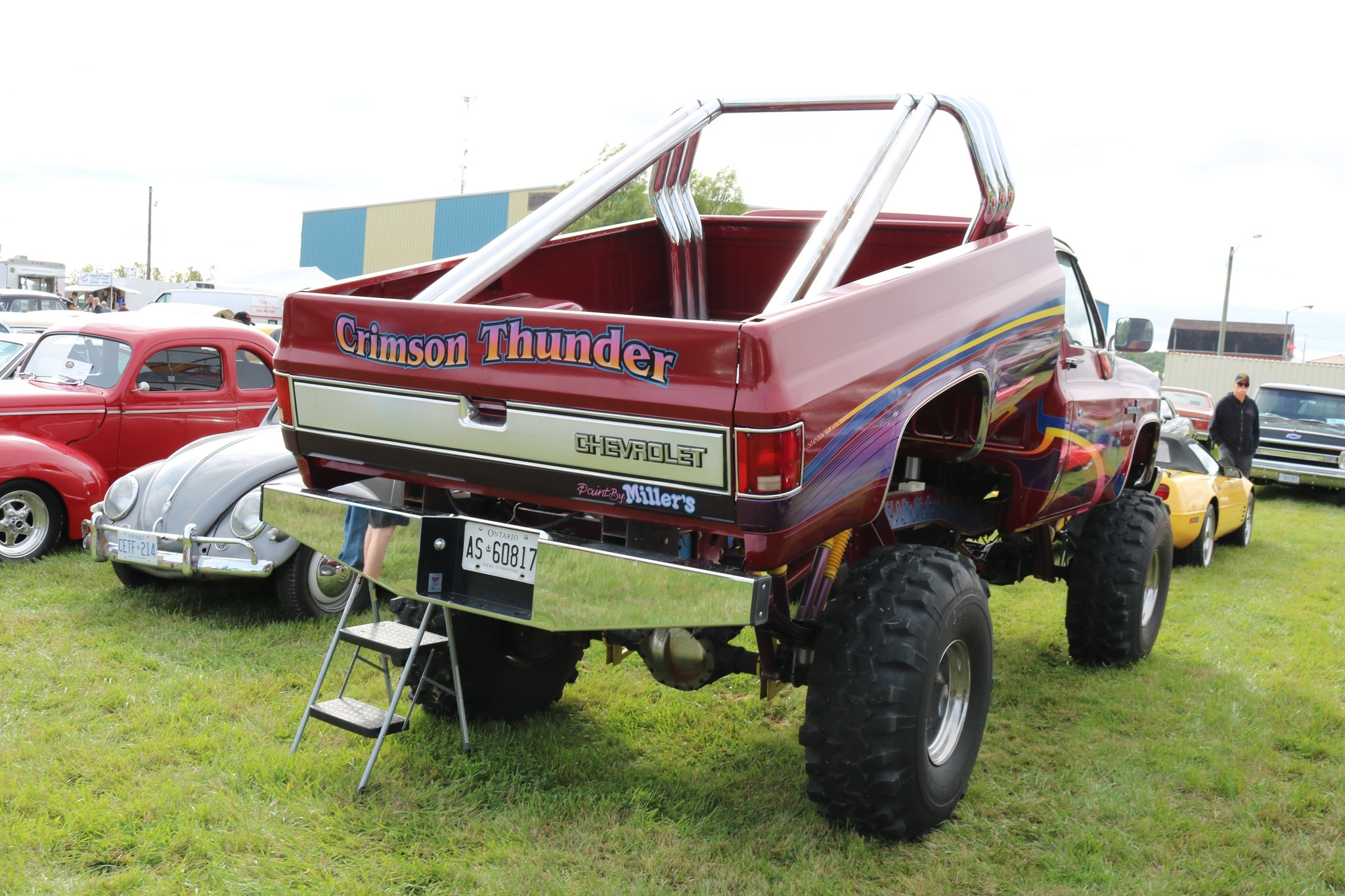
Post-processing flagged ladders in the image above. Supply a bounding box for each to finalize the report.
[289,574,472,797]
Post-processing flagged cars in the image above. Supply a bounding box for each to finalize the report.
[0,290,281,568]
[1154,387,1256,569]
[82,397,387,631]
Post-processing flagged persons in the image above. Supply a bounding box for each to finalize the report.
[337,505,410,581]
[56,291,130,314]
[1207,373,1260,480]
[232,311,281,360]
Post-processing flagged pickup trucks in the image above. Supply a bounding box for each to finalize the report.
[260,91,1174,848]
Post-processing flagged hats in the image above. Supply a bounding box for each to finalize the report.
[233,311,255,326]
[1234,373,1250,383]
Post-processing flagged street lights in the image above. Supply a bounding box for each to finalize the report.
[1281,305,1312,361]
[1217,234,1262,357]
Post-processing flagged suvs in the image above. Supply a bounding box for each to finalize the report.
[1249,381,1344,490]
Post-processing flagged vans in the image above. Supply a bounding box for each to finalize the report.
[148,291,283,326]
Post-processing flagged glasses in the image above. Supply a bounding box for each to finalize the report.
[1236,382,1249,388]
